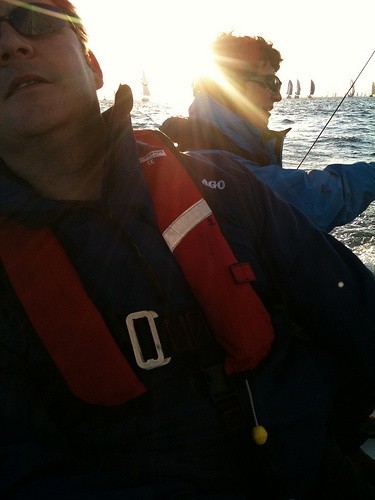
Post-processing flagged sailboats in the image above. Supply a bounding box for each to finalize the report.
[308,80,315,98]
[141,71,150,102]
[347,80,355,97]
[294,78,301,99]
[370,82,375,97]
[286,80,292,99]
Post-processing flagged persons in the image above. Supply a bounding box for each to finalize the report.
[0,0,375,500]
[159,33,375,235]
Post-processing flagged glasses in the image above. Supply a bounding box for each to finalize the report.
[0,3,81,42]
[238,74,282,94]
